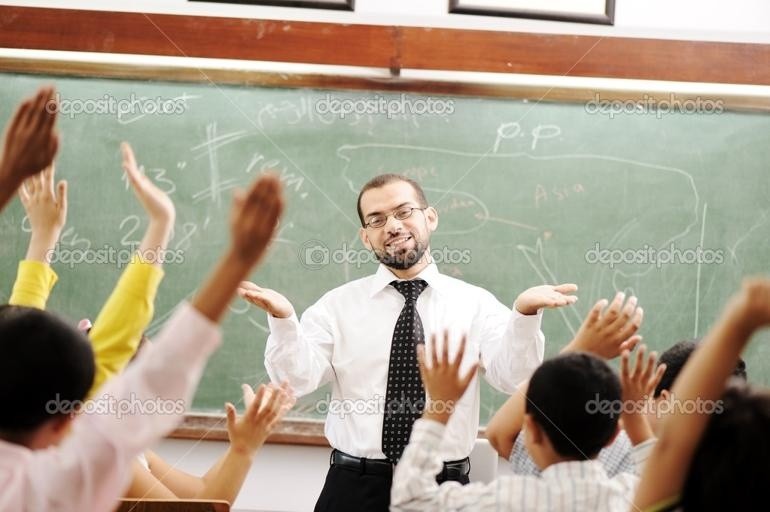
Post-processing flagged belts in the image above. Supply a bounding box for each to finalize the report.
[333,452,471,476]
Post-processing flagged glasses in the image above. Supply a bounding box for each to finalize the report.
[364,207,424,229]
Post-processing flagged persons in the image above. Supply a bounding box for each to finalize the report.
[0,84,300,511]
[236,173,579,512]
[389,273,770,512]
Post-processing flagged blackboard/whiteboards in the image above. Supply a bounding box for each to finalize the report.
[0,47,770,446]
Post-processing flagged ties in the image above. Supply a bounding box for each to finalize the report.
[381,278,428,466]
